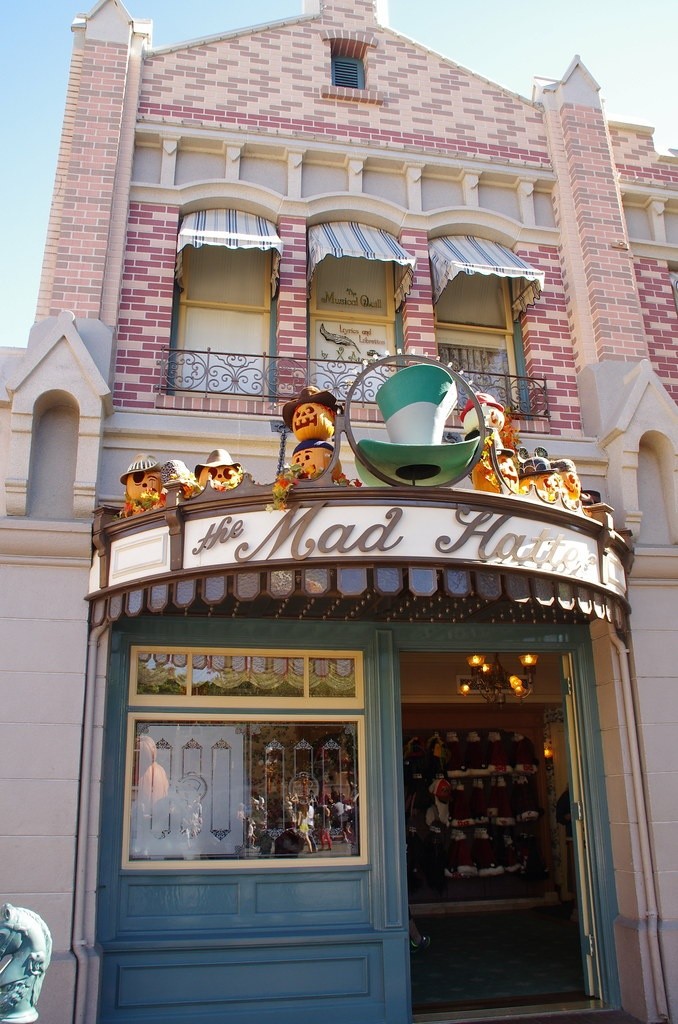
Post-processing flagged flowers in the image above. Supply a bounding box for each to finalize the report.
[111,467,252,523]
[265,464,362,513]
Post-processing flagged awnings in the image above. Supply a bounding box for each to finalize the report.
[175,209,284,295]
[429,235,545,324]
[306,222,418,313]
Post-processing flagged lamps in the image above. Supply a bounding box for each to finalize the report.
[459,652,539,714]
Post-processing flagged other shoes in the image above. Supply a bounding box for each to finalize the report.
[410,936,431,953]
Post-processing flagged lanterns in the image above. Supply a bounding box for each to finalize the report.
[282,386,342,487]
[466,428,581,504]
[119,448,245,504]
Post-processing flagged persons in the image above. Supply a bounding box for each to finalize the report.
[130,785,357,860]
[554,783,582,921]
[407,868,432,954]
